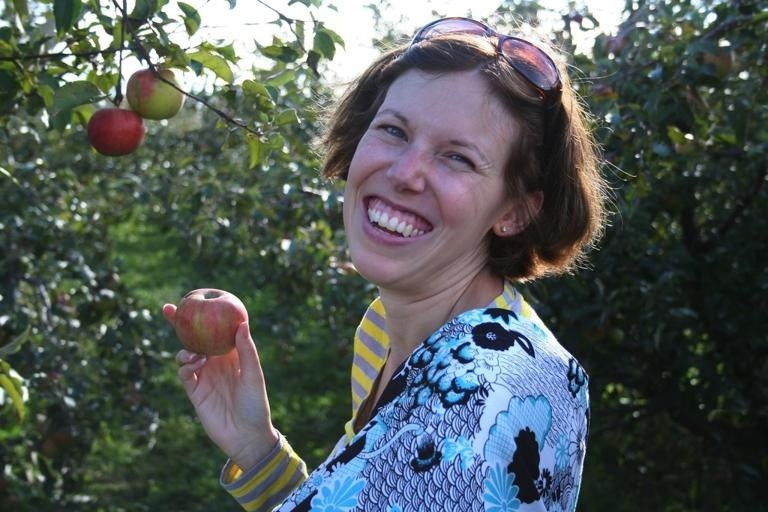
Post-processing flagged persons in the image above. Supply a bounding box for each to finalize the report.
[160,16,609,509]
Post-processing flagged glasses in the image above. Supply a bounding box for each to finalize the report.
[411,16,559,92]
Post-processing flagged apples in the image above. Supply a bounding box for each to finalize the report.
[87,108,146,156]
[174,288,250,356]
[126,69,182,120]
